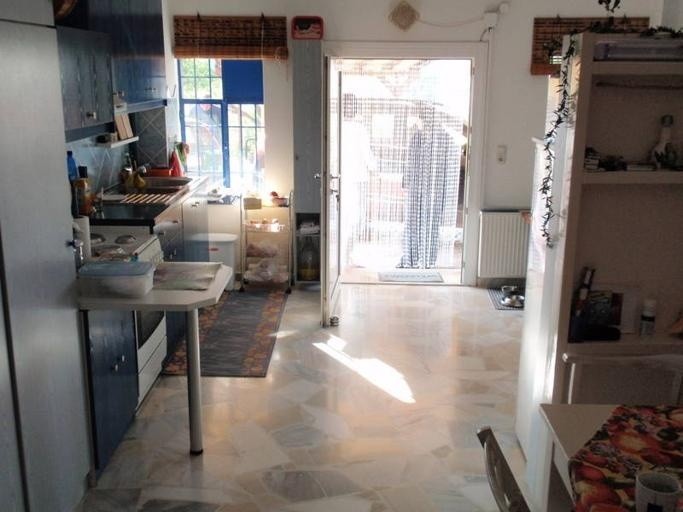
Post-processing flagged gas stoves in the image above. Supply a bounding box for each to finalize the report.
[82,231,157,260]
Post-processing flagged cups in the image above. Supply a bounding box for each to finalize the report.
[633,470,679,511]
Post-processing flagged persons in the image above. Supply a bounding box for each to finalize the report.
[395,107,451,269]
[189,92,220,171]
[329,92,378,270]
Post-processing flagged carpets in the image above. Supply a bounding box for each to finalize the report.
[489,288,523,310]
[162,287,289,377]
[378,269,443,282]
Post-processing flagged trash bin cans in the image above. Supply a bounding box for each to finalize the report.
[190,232,239,292]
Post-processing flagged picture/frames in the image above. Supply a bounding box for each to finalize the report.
[291,16,324,39]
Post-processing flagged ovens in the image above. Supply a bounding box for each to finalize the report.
[134,251,166,399]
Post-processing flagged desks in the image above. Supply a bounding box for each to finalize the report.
[539,403,683,512]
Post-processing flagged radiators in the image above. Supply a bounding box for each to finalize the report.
[478,208,531,278]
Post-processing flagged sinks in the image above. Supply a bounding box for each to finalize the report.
[109,187,181,195]
[142,177,193,187]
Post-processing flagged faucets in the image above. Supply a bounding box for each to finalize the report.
[134,166,147,180]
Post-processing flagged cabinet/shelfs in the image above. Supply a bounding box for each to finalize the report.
[0,0,93,512]
[152,176,210,356]
[293,213,321,283]
[57,0,166,132]
[83,307,139,471]
[515,34,683,461]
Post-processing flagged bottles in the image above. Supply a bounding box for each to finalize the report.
[248,217,283,233]
[650,115,678,170]
[640,297,656,338]
[67,149,77,182]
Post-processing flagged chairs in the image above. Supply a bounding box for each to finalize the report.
[561,352,683,404]
[476,426,532,512]
